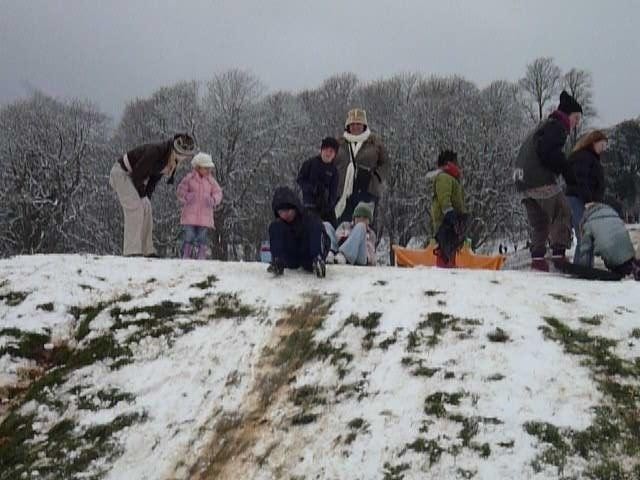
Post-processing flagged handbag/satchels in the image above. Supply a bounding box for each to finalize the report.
[436,212,471,261]
[355,168,372,202]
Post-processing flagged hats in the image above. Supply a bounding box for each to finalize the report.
[345,108,367,130]
[558,91,581,113]
[174,134,197,155]
[352,201,372,225]
[192,152,215,168]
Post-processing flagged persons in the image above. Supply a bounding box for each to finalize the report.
[565,129,610,265]
[109,132,195,255]
[515,90,582,271]
[430,149,473,268]
[297,138,338,216]
[265,189,330,277]
[323,203,378,264]
[334,108,392,230]
[574,202,640,280]
[176,153,222,257]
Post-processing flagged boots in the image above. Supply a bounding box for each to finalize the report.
[198,241,207,259]
[182,241,193,258]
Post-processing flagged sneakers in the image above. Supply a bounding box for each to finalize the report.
[268,261,283,274]
[313,256,325,277]
[335,252,346,264]
[326,250,335,264]
